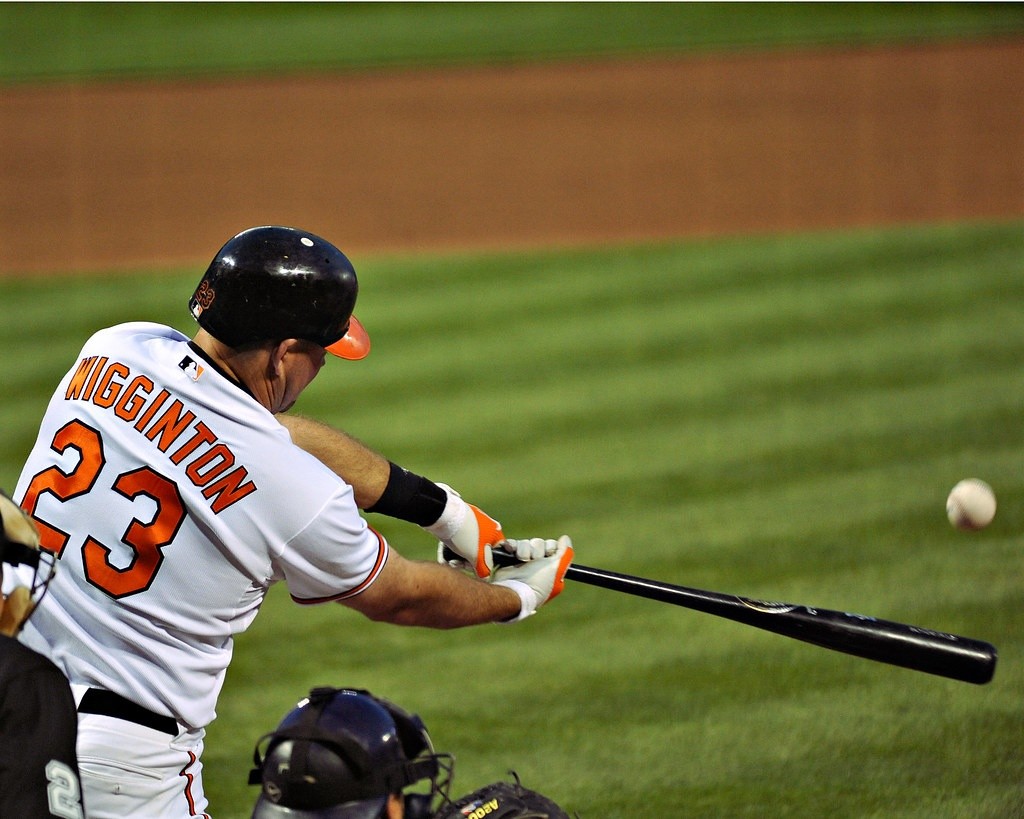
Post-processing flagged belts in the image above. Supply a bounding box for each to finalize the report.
[78,687,179,738]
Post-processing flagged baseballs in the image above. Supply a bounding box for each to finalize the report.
[945,474,997,532]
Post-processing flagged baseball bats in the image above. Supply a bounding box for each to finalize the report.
[440,540,1000,687]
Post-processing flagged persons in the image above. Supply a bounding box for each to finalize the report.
[245,685,579,817]
[0,489,88,819]
[14,226,574,818]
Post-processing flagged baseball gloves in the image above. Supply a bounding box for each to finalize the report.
[445,768,580,819]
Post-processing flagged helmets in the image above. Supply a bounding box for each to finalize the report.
[188,224,371,361]
[251,687,412,819]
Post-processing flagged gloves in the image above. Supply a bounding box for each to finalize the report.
[416,481,508,583]
[489,534,574,623]
